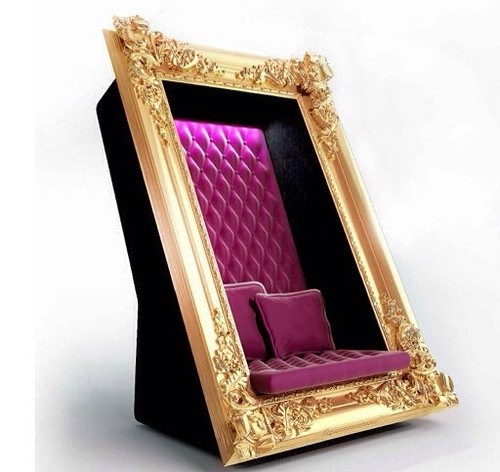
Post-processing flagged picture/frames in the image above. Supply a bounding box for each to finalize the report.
[100,13,460,470]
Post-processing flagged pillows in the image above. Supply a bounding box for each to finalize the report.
[252,289,337,360]
[222,280,274,362]
